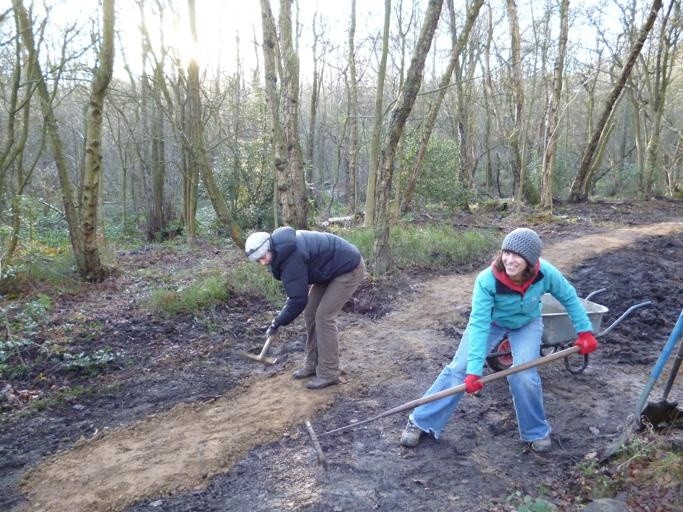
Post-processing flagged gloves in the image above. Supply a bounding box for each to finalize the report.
[266,318,278,338]
[464,375,483,393]
[574,332,598,355]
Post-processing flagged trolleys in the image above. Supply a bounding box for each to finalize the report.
[486,288,651,374]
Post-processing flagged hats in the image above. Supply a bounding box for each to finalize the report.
[502,228,543,265]
[244,231,271,263]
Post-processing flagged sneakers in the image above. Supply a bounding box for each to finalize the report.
[291,365,316,379]
[400,418,421,448]
[305,376,339,389]
[528,435,552,453]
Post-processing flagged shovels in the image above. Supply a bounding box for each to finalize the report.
[600,305,683,464]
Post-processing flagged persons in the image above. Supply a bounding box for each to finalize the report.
[400,226,599,452]
[245,227,365,389]
